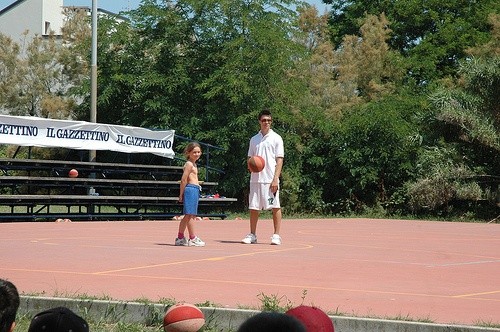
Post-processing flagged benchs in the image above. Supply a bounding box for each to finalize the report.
[0,115,238,221]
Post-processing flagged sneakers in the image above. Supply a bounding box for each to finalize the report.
[241,233,257,244]
[270,234,281,244]
[188,238,205,247]
[175,237,188,245]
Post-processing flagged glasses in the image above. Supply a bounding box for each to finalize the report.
[260,119,272,122]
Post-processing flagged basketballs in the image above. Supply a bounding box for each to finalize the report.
[286,306,334,332]
[248,156,265,172]
[165,302,205,332]
[69,169,78,177]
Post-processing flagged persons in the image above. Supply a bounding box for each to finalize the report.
[0,278,89,332]
[175,142,206,246]
[241,109,285,245]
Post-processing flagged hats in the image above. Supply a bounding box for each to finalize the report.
[285,305,335,332]
[30,307,89,332]
